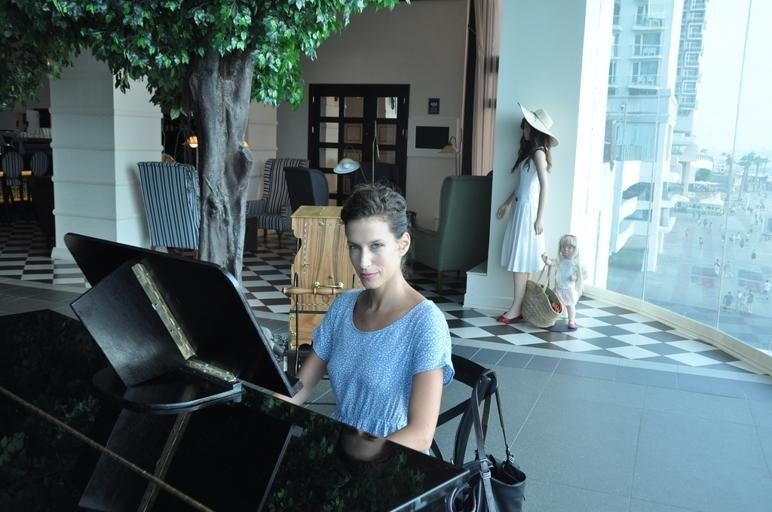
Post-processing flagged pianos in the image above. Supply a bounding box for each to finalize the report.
[0,310,471,511]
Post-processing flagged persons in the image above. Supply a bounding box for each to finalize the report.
[272,184,455,456]
[497,101,559,323]
[541,235,583,328]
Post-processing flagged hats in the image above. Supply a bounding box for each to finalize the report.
[332,158,359,174]
[517,101,559,147]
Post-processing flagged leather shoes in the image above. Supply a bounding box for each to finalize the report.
[497,311,522,323]
[568,320,575,329]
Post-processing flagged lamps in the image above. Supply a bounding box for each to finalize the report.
[333,157,368,184]
[439,136,460,154]
[182,134,198,149]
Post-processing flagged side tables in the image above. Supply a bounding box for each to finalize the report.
[244,214,258,253]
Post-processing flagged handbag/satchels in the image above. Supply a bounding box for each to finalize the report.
[446,453,527,512]
[520,262,564,329]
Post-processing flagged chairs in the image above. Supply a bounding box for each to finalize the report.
[245,158,310,251]
[2,151,24,203]
[282,166,329,252]
[407,176,493,294]
[137,162,203,261]
[27,151,52,204]
[431,353,499,463]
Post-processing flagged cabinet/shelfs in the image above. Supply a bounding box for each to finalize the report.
[287,206,365,353]
